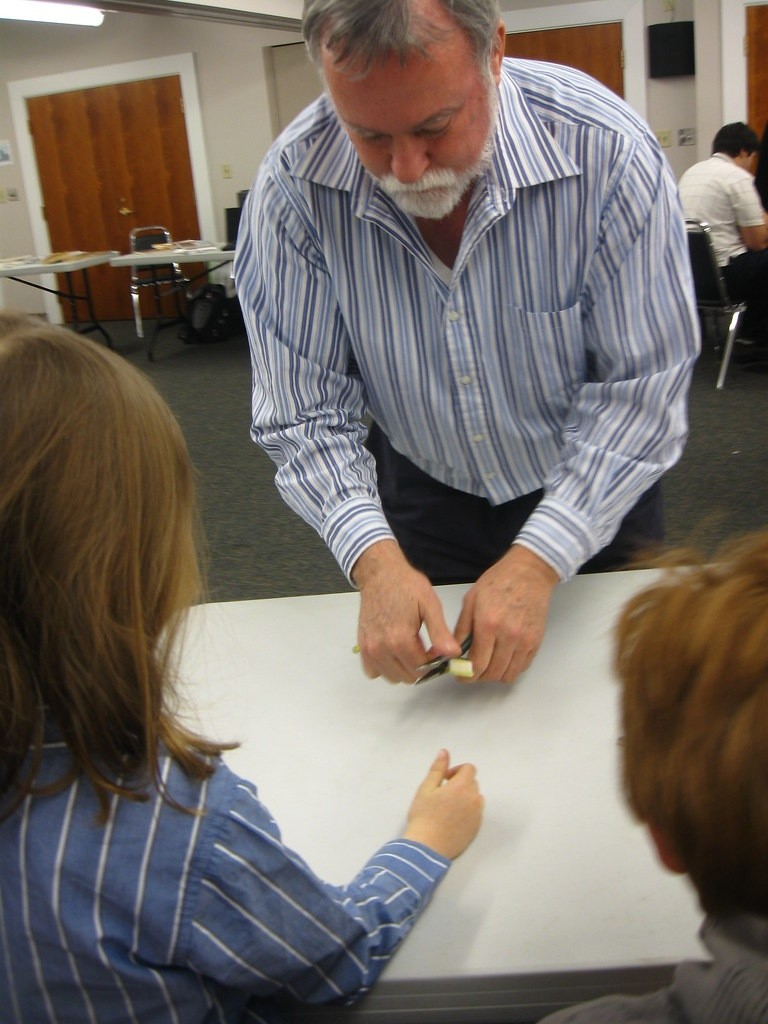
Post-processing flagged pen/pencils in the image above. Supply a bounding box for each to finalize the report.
[412,632,478,692]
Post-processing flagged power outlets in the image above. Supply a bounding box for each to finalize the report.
[655,129,671,147]
[679,128,696,146]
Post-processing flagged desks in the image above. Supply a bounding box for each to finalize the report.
[158,566,714,1024]
[110,241,235,361]
[0,249,122,352]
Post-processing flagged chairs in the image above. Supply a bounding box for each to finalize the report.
[680,216,768,392]
[132,226,191,337]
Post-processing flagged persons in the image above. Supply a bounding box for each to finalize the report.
[222,1,702,691]
[0,313,480,1024]
[677,120,768,363]
[531,521,768,1024]
